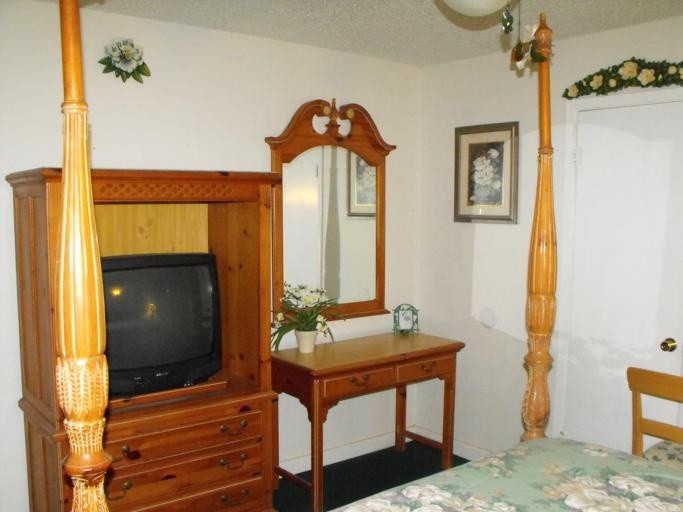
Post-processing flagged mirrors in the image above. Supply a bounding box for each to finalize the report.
[265,98,397,325]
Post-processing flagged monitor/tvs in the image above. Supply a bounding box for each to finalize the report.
[99,253,222,394]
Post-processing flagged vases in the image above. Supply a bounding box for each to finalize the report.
[293,330,319,353]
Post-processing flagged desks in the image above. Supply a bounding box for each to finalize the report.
[273,332,467,512]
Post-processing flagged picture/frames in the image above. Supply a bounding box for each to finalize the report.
[454,121,519,224]
[347,150,376,217]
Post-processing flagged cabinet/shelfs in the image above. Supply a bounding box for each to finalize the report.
[6,168,280,512]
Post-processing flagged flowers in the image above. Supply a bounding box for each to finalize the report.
[272,281,348,353]
[563,56,683,100]
[97,38,151,84]
